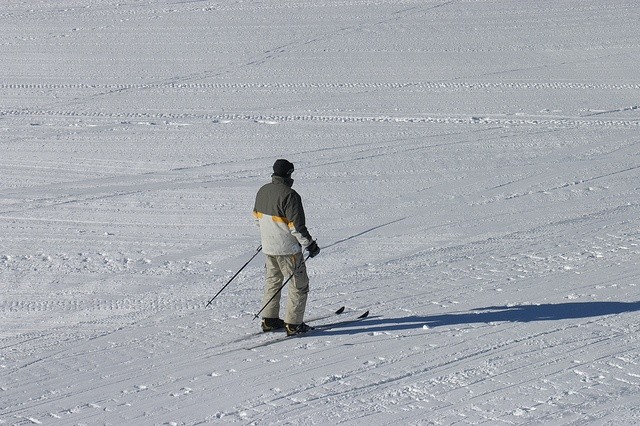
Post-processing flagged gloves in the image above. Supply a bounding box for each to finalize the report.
[305,240,320,258]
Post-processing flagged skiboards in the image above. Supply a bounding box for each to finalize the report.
[233,306,369,351]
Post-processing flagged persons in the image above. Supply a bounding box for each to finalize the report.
[251,159,322,339]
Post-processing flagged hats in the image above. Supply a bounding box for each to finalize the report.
[273,159,294,177]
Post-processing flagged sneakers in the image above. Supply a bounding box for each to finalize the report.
[289,322,315,333]
[265,317,285,328]
